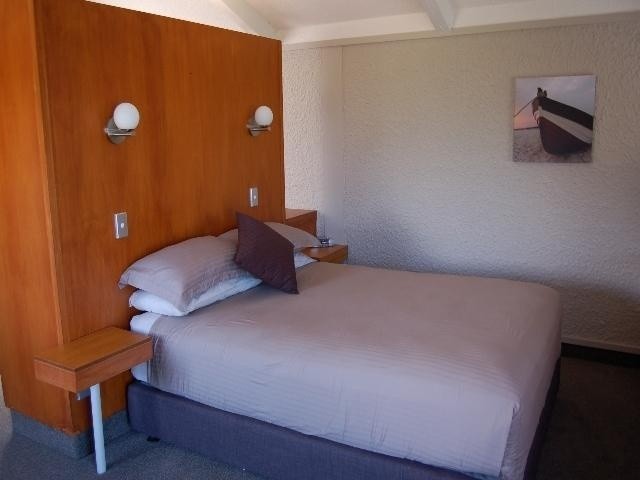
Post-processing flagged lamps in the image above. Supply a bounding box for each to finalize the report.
[103,101,142,146]
[246,104,276,138]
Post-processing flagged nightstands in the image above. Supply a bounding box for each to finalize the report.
[285,207,349,264]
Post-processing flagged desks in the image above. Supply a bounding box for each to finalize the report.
[33,323,154,474]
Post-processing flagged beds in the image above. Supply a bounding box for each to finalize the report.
[124,256,563,480]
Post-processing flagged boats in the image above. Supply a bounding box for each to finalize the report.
[531,86,593,156]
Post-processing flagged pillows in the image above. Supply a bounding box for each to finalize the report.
[118,210,326,318]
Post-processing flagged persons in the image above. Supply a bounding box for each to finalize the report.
[537,87,548,98]
